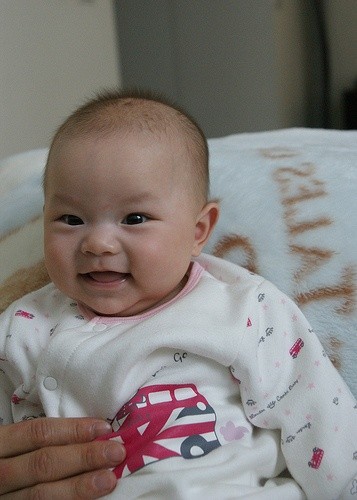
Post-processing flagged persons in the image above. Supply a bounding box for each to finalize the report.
[0,87,357,500]
[0,418,130,500]
